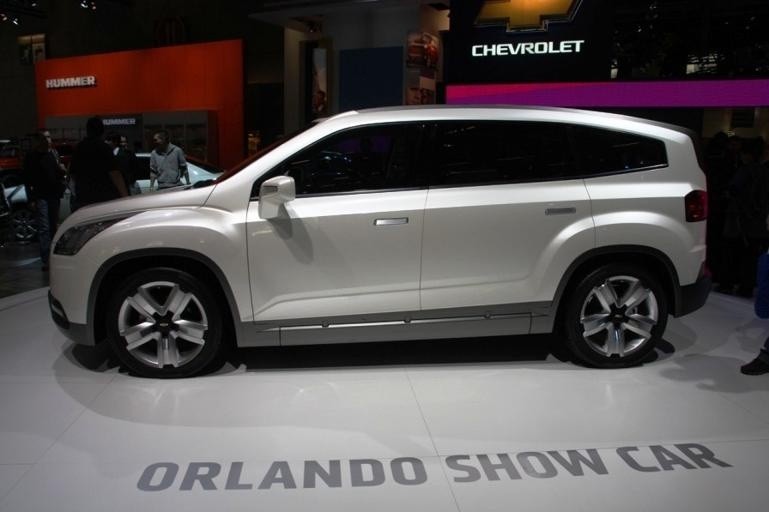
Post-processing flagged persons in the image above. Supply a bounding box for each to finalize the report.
[120,135,128,150]
[705,132,768,300]
[104,133,140,196]
[40,128,64,170]
[149,130,192,192]
[68,116,130,215]
[407,87,421,105]
[739,333,768,375]
[23,128,67,273]
[310,89,327,119]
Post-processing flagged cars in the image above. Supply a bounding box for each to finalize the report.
[48,104,712,379]
[0,139,227,241]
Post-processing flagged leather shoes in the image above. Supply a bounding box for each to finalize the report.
[742,349,769,375]
